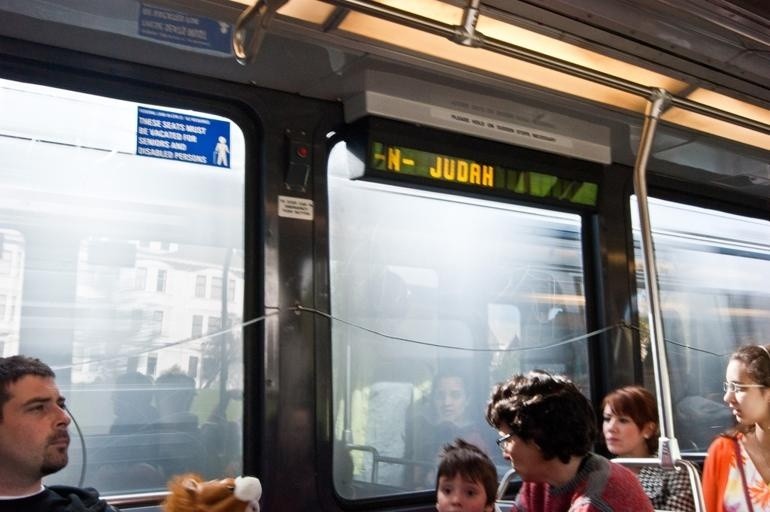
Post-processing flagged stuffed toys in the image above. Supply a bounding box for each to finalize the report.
[160,474,263,512]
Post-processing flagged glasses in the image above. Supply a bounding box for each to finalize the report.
[722,381,765,395]
[496,430,516,450]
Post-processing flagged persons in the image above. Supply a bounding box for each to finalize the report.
[138,371,202,482]
[0,355,122,512]
[92,370,160,497]
[701,344,770,512]
[430,372,491,460]
[601,384,696,512]
[484,369,655,512]
[435,438,498,512]
